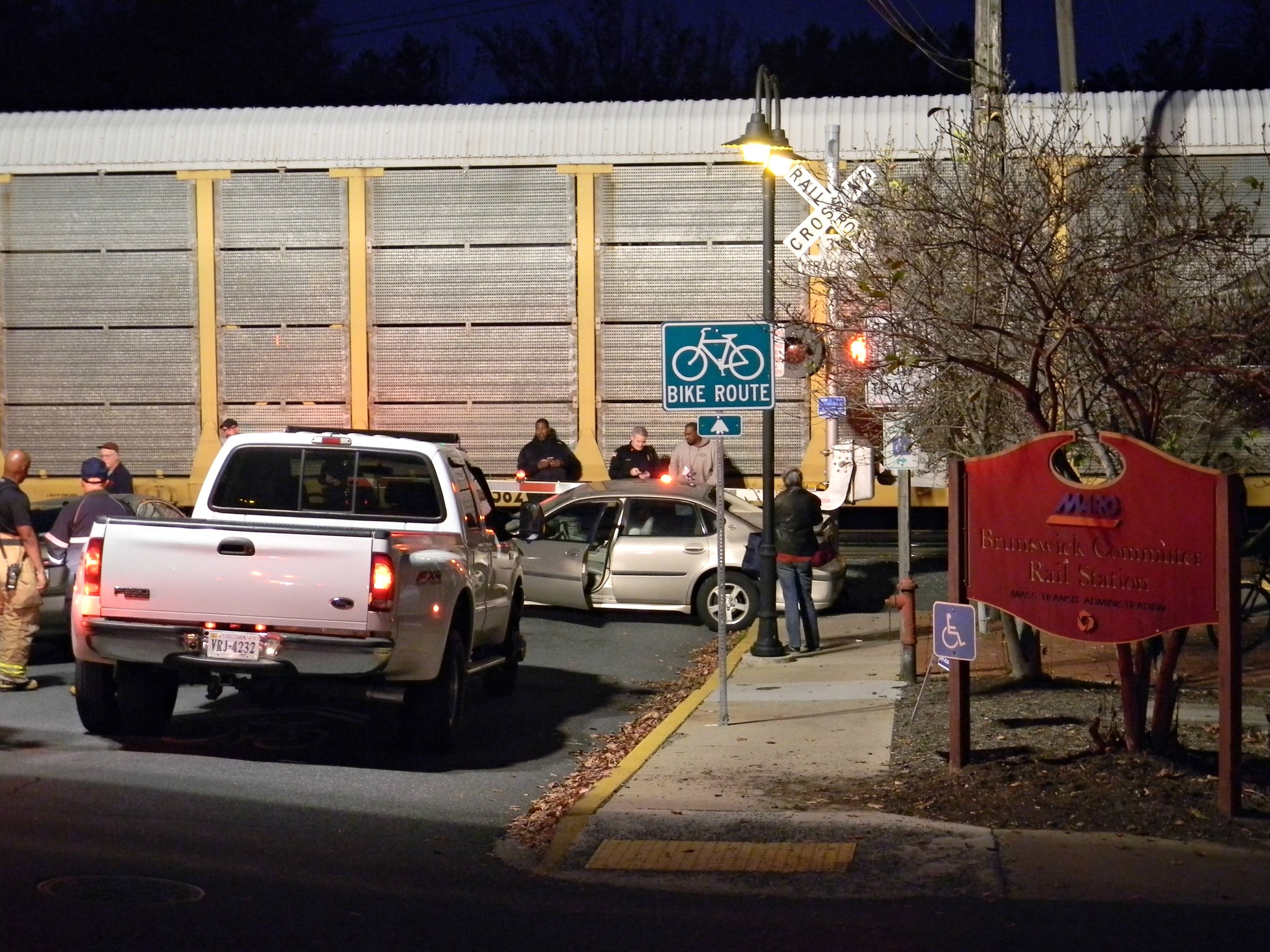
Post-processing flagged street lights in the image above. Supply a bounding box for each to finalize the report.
[722,63,821,666]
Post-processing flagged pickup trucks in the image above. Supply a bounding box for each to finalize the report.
[70,423,544,755]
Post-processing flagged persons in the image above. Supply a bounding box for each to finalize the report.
[773,467,824,654]
[609,426,660,508]
[1217,452,1247,556]
[45,457,136,698]
[669,422,716,515]
[318,458,362,511]
[0,451,50,692]
[219,418,239,439]
[96,441,133,494]
[518,417,581,504]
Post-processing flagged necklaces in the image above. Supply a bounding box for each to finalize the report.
[688,436,701,480]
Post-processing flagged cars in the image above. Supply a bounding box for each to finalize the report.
[30,491,187,628]
[498,476,848,630]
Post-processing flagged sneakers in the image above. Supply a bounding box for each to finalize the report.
[783,644,800,654]
[803,645,820,650]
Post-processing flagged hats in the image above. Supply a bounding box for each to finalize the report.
[97,441,119,453]
[81,457,107,484]
[220,418,238,428]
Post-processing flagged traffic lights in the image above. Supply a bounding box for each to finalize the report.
[838,328,867,370]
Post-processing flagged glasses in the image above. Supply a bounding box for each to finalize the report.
[222,426,236,432]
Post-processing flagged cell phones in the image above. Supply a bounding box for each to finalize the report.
[637,470,646,475]
[546,457,554,461]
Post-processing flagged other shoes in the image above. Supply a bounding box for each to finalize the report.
[0,680,37,691]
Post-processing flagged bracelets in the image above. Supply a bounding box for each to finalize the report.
[36,570,46,574]
